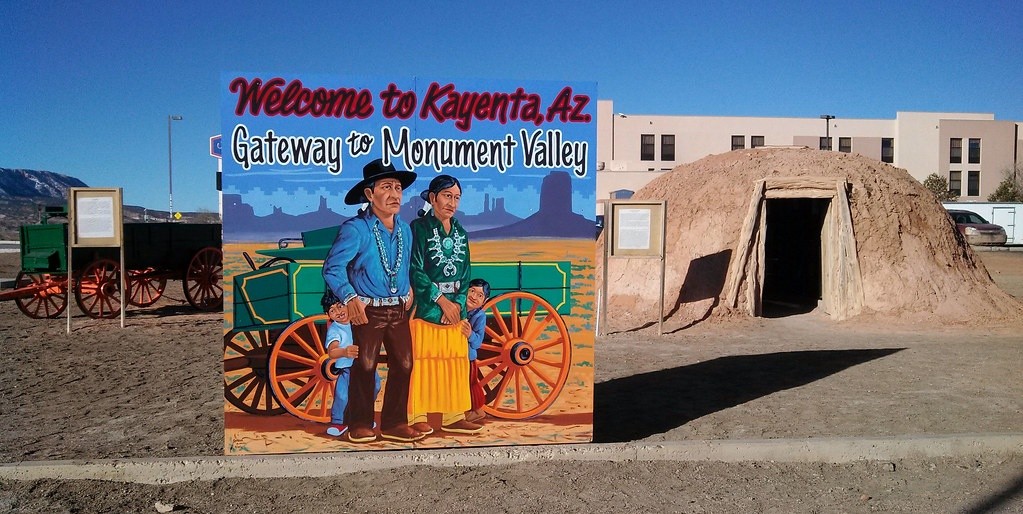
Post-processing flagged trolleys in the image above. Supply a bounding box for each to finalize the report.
[0,202,225,319]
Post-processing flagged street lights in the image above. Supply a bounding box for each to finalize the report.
[167,115,183,220]
[820,114,835,152]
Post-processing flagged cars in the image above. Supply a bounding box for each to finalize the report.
[947,208,1008,246]
